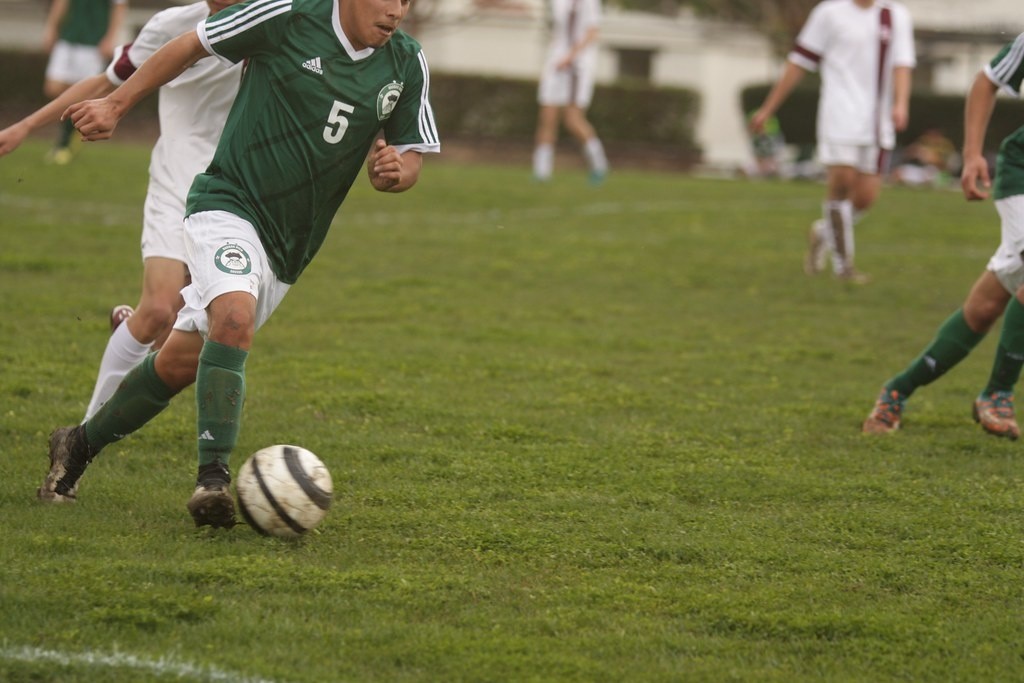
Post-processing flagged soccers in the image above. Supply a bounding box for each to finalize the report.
[235,444,334,540]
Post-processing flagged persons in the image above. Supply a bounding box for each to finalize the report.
[864,33,1024,438]
[531,0,610,182]
[749,0,916,279]
[0,0,441,532]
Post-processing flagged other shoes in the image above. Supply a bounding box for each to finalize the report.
[110,305,134,330]
[805,217,831,277]
[830,265,869,284]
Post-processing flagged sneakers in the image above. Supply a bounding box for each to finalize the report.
[186,456,236,529]
[862,381,906,434]
[37,428,90,502]
[972,390,1020,440]
